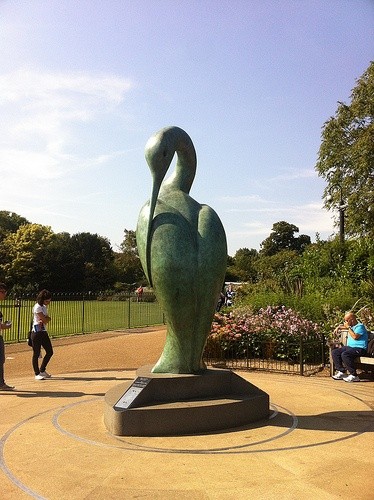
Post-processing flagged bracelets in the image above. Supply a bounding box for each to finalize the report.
[348,327,351,329]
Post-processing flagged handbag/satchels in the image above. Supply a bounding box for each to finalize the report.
[27,331,32,346]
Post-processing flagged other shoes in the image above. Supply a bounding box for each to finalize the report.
[0,383,15,391]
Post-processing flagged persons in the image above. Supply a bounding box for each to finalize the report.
[138,286,143,302]
[0,283,15,390]
[31,290,54,379]
[219,285,234,307]
[332,313,369,382]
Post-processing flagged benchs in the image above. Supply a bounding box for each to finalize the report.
[330,328,374,377]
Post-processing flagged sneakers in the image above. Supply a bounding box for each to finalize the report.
[35,375,44,380]
[40,371,51,378]
[343,375,360,382]
[332,371,348,380]
